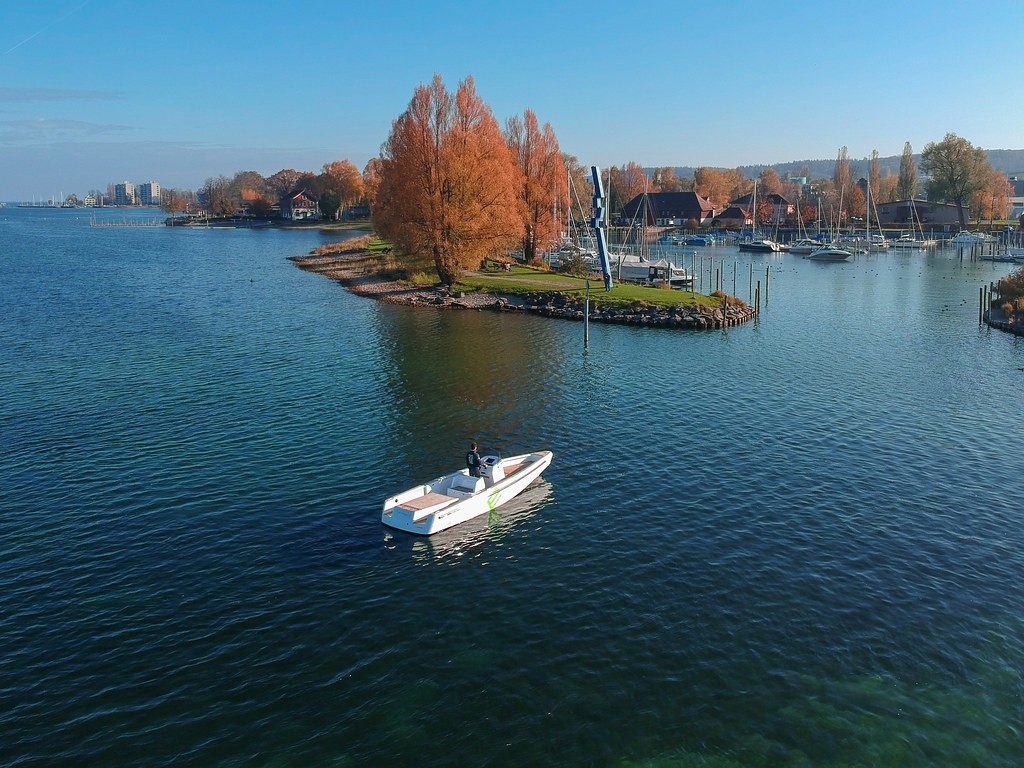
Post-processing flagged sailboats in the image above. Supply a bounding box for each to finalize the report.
[511,168,1024,285]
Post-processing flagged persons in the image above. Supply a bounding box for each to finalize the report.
[502,261,512,272]
[465,442,488,477]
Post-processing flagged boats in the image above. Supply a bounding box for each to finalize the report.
[382,450,554,535]
